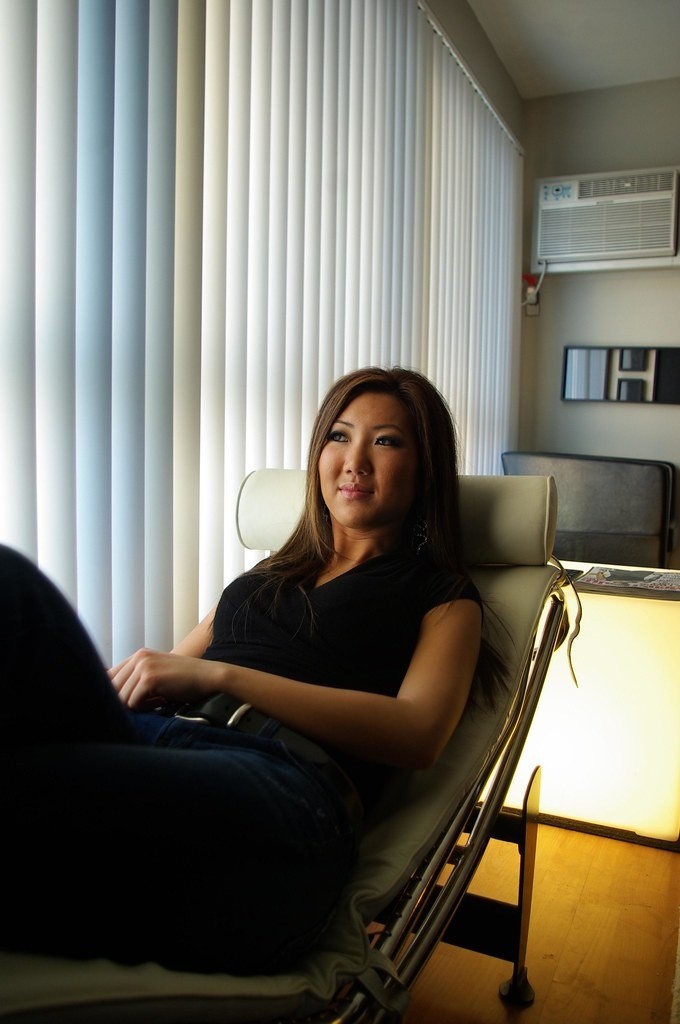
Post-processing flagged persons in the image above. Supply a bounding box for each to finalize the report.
[0,367,516,973]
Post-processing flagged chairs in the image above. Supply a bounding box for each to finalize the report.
[0,468,584,1024]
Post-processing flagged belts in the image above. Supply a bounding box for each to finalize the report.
[164,689,365,831]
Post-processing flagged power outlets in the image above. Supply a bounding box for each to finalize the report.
[526,287,540,316]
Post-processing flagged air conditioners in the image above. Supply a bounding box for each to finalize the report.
[537,169,679,264]
